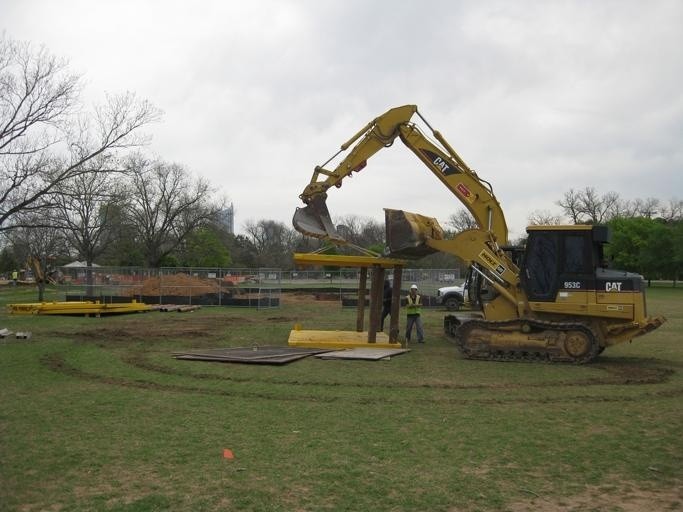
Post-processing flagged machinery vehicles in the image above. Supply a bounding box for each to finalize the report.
[16,255,58,287]
[380,205,666,366]
[292,103,525,344]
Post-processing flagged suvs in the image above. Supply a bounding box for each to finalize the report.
[436,277,472,312]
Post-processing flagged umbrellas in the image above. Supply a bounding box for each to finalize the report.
[62,261,88,280]
[79,260,101,281]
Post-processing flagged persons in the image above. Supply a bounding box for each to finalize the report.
[380,280,392,332]
[404,283,427,344]
[10,269,17,286]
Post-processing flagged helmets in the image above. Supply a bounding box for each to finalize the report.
[411,285,418,289]
